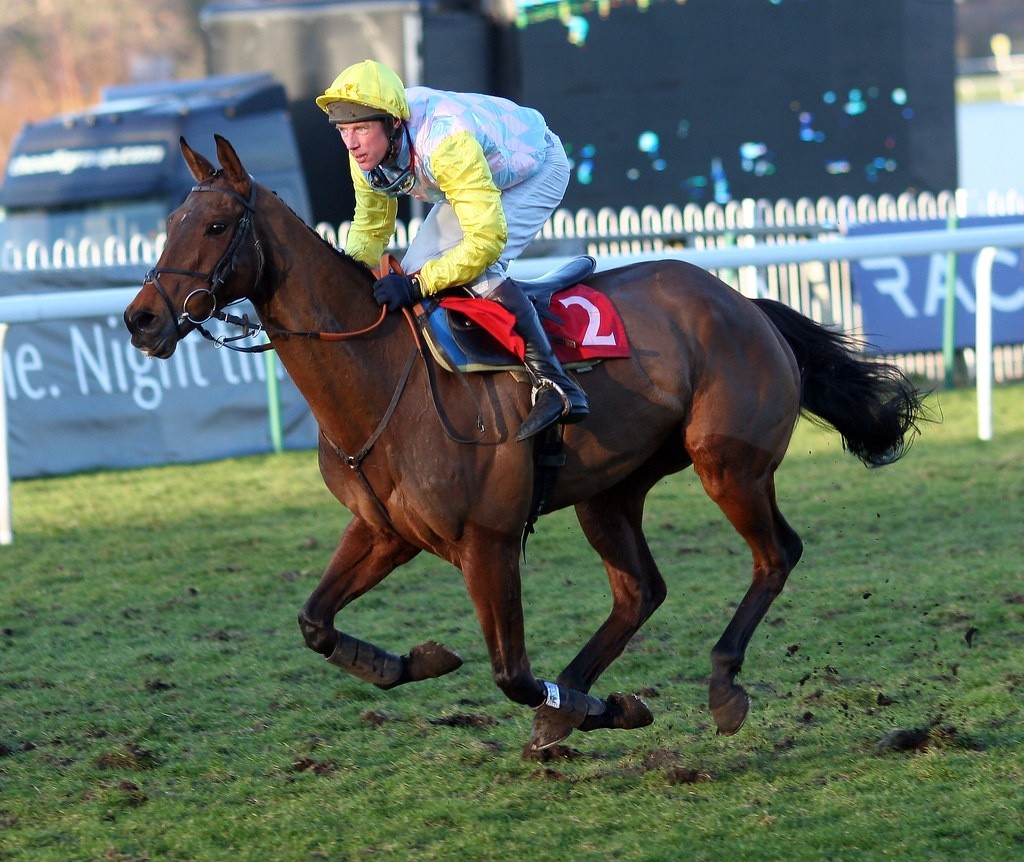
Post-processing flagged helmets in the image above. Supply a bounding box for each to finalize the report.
[329,101,392,122]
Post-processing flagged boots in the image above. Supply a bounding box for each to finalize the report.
[485,277,589,443]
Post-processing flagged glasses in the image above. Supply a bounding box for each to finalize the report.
[368,119,417,198]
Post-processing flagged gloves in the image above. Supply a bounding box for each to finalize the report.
[373,273,424,313]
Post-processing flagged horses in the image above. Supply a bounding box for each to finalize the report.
[122,125,946,766]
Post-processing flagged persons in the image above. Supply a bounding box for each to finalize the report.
[316,60,589,442]
